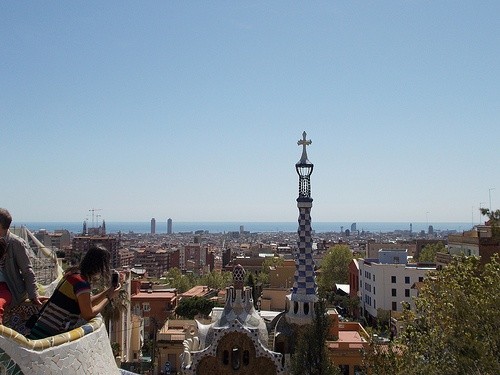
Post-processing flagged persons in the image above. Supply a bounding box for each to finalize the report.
[26,246,122,341]
[1,207,42,325]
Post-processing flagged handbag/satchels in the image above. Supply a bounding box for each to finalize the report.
[25,314,41,329]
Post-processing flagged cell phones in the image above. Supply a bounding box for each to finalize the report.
[112,273,120,288]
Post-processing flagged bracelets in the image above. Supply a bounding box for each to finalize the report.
[106,295,111,303]
